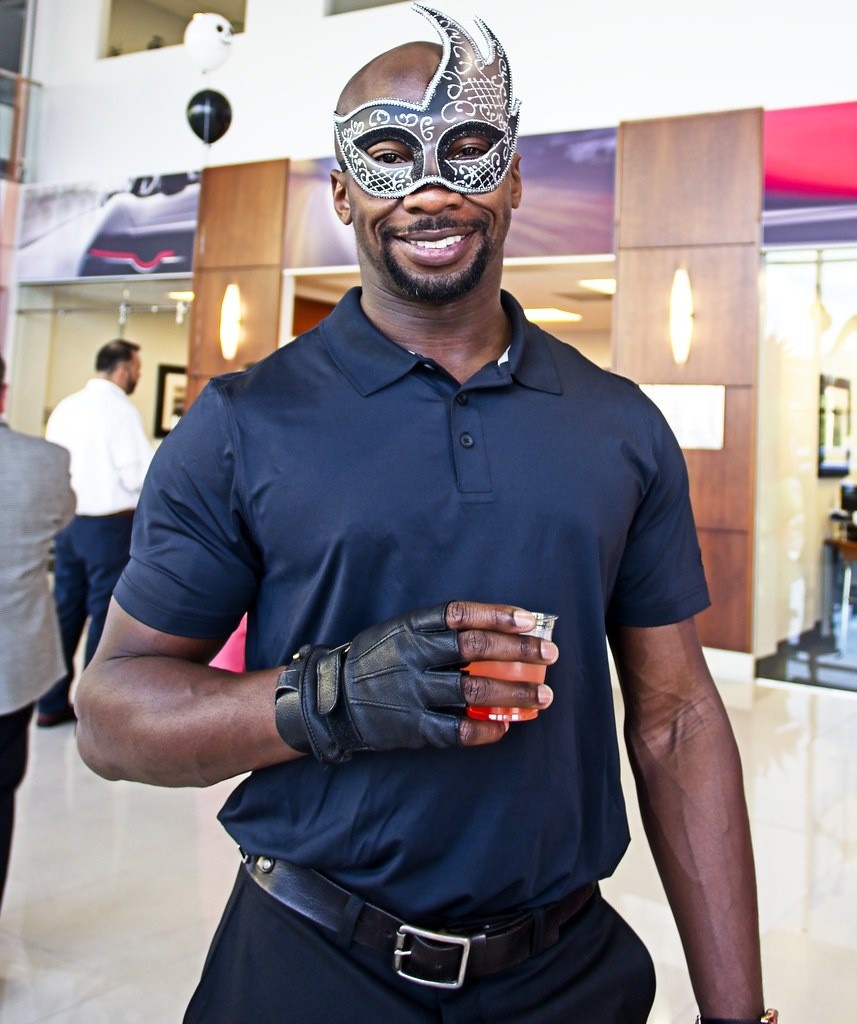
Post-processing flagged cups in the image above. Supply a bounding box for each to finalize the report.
[459,611,559,721]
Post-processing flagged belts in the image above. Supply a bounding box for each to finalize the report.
[240,845,599,989]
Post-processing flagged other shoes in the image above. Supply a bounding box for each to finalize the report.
[38,704,75,727]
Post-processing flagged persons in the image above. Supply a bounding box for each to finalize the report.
[0,359,77,929]
[73,42,773,1024]
[37,339,151,728]
